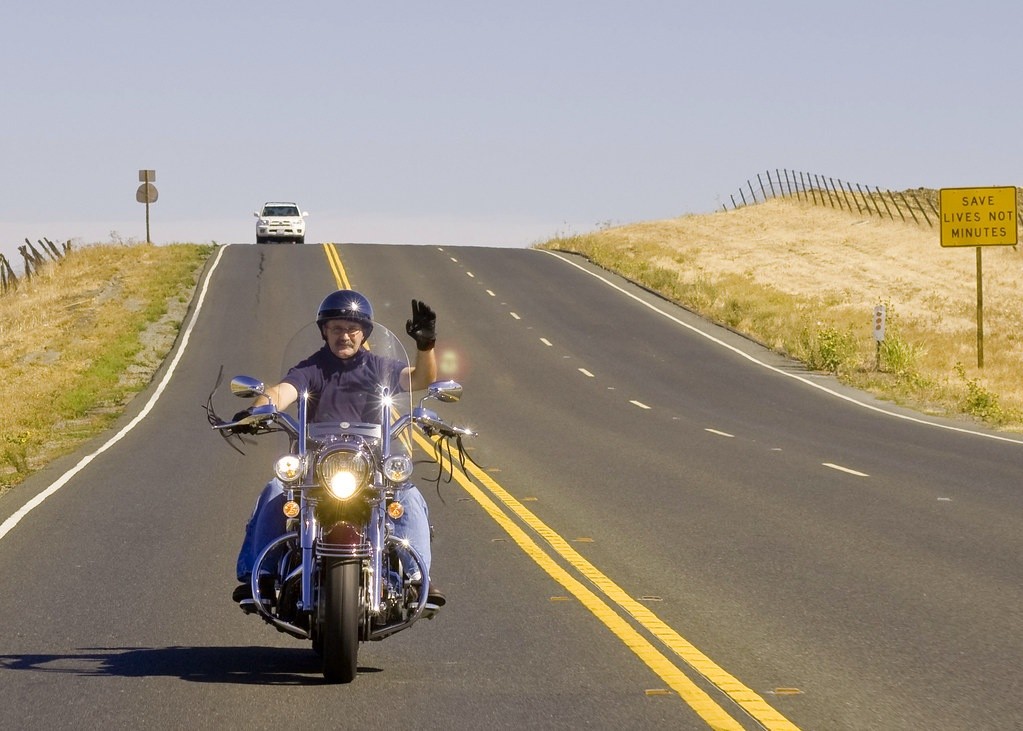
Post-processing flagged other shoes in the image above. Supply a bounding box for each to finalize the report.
[405,577,446,607]
[232,578,276,603]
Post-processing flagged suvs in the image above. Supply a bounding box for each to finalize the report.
[253,201,309,244]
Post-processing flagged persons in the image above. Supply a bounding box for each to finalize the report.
[231,289,447,608]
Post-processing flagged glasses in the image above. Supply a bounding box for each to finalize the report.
[325,326,364,336]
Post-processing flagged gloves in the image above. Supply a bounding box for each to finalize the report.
[231,407,259,436]
[406,299,436,351]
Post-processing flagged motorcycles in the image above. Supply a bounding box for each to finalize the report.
[210,320,478,685]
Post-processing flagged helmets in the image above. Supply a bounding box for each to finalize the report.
[316,289,374,344]
